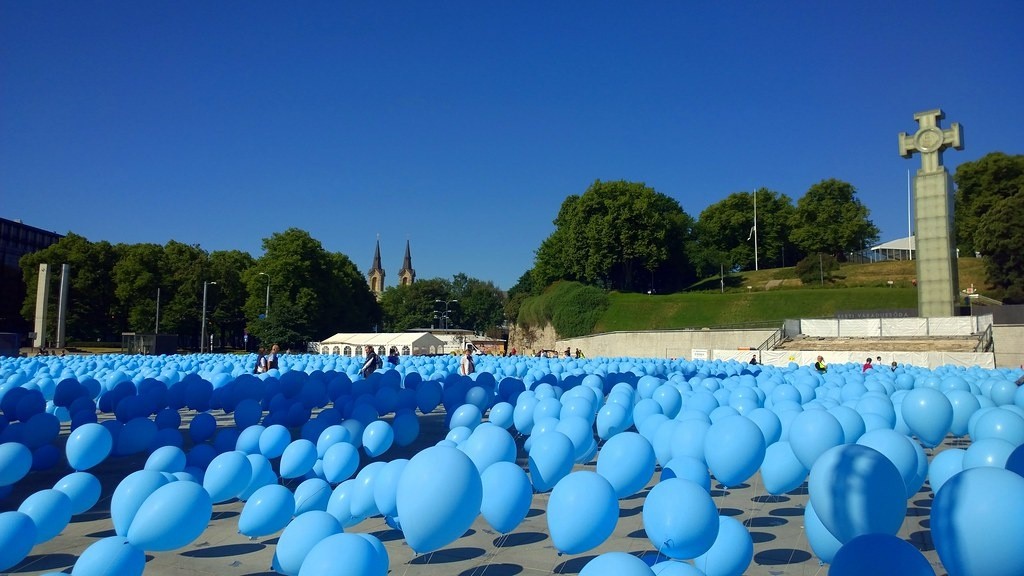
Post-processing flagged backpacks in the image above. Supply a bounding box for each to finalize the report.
[370,353,383,369]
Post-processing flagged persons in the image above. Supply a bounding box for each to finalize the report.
[479,344,487,355]
[891,362,898,371]
[815,356,828,371]
[254,348,267,373]
[862,358,873,372]
[358,345,383,379]
[541,349,547,357]
[876,356,882,365]
[60,351,66,356]
[138,348,142,354]
[553,351,558,357]
[510,347,516,356]
[749,355,757,364]
[461,350,474,375]
[286,348,291,354]
[52,349,56,355]
[579,350,584,358]
[387,349,399,366]
[268,345,280,369]
[576,348,580,358]
[564,347,570,355]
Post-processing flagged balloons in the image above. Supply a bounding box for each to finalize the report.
[643,478,720,559]
[0,352,1023,576]
[808,444,907,547]
[396,446,483,555]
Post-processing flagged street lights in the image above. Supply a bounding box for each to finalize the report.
[434,310,452,330]
[435,299,458,334]
[259,272,270,318]
[200,281,217,355]
